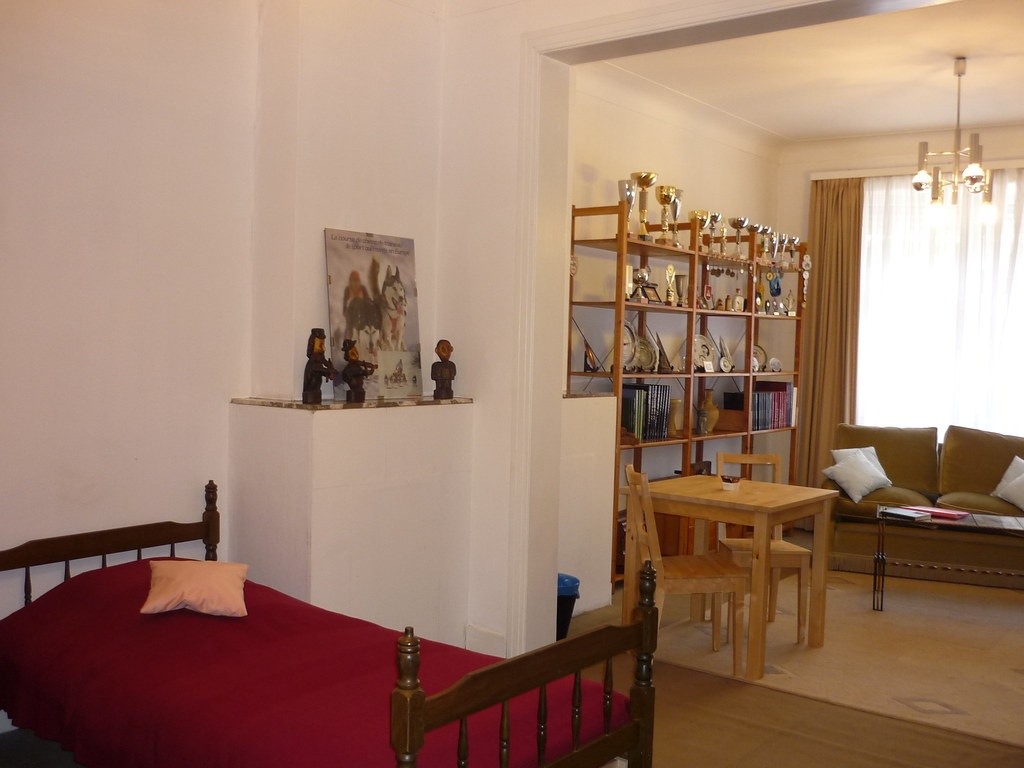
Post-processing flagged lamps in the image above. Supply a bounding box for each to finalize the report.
[911,56,996,207]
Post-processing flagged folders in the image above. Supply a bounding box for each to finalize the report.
[902,506,970,520]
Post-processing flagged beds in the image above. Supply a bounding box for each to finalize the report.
[0,480,659,768]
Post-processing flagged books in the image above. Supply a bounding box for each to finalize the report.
[723,380,797,429]
[622,383,672,442]
[880,506,969,521]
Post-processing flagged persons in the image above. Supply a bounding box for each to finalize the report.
[342,339,373,390]
[303,328,337,390]
[431,340,456,392]
[343,270,369,317]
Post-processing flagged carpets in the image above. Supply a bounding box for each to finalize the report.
[654,571,1024,749]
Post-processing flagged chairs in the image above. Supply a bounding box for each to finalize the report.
[626,462,749,678]
[716,451,812,645]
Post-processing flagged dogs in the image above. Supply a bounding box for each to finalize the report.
[345,263,408,351]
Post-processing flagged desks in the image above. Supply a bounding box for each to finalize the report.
[617,474,840,681]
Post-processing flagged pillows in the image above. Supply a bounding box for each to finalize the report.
[831,446,892,489]
[990,455,1024,497]
[139,559,249,618]
[997,473,1024,511]
[823,450,893,504]
[940,425,1024,498]
[834,423,939,495]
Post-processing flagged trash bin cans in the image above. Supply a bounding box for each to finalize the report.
[555,573,580,641]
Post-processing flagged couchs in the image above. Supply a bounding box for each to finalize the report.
[811,443,1024,591]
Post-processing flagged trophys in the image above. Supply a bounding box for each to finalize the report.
[615,180,637,239]
[630,172,658,243]
[627,263,688,307]
[688,210,711,253]
[706,210,722,255]
[718,216,800,265]
[655,185,675,247]
[669,189,683,250]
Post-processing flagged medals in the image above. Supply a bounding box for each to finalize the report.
[697,252,797,317]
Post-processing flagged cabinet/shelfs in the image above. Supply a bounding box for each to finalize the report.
[568,200,809,595]
